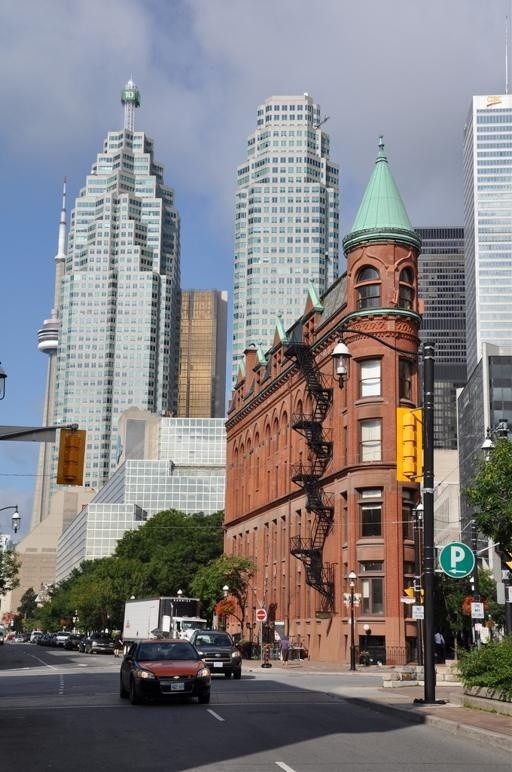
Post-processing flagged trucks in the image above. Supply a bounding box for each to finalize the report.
[121,596,210,656]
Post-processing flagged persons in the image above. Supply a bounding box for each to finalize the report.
[293,633,303,661]
[4,631,17,648]
[279,635,291,666]
[113,635,123,658]
[474,613,505,651]
[433,629,447,662]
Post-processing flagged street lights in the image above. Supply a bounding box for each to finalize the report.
[0,506,21,533]
[176,588,182,597]
[222,573,231,596]
[349,570,356,670]
[330,328,436,703]
[481,419,509,449]
[0,362,7,400]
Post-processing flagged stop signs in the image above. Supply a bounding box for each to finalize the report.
[256,609,267,622]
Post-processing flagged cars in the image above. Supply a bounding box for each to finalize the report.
[0,632,117,654]
[120,629,242,705]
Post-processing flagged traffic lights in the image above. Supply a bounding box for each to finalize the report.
[504,549,512,573]
[403,586,424,608]
